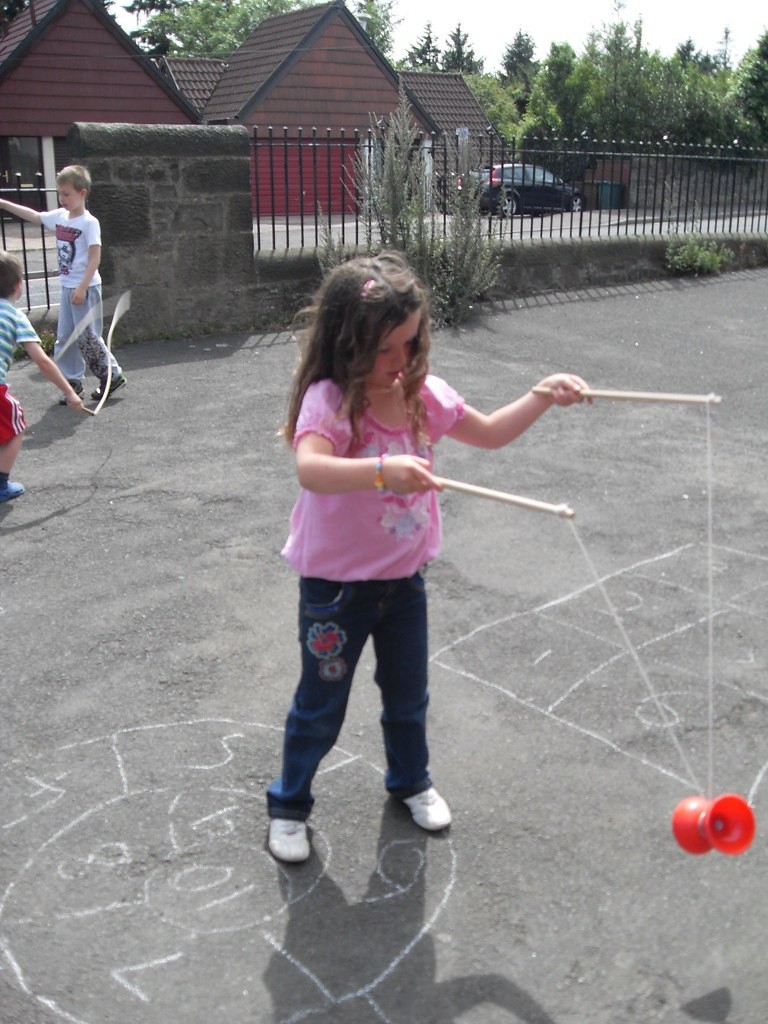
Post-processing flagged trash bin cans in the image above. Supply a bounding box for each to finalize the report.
[583,180,601,210]
[598,181,624,209]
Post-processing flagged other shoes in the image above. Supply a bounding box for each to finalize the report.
[0,481,25,502]
[60,384,84,405]
[91,374,127,399]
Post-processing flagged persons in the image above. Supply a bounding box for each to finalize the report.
[265,254,592,861]
[0,250,83,501]
[0,165,126,405]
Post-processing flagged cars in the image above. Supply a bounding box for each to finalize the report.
[452,162,588,218]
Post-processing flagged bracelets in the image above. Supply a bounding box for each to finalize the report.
[375,453,390,493]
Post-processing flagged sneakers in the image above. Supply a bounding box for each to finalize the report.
[403,785,452,831]
[269,818,310,862]
